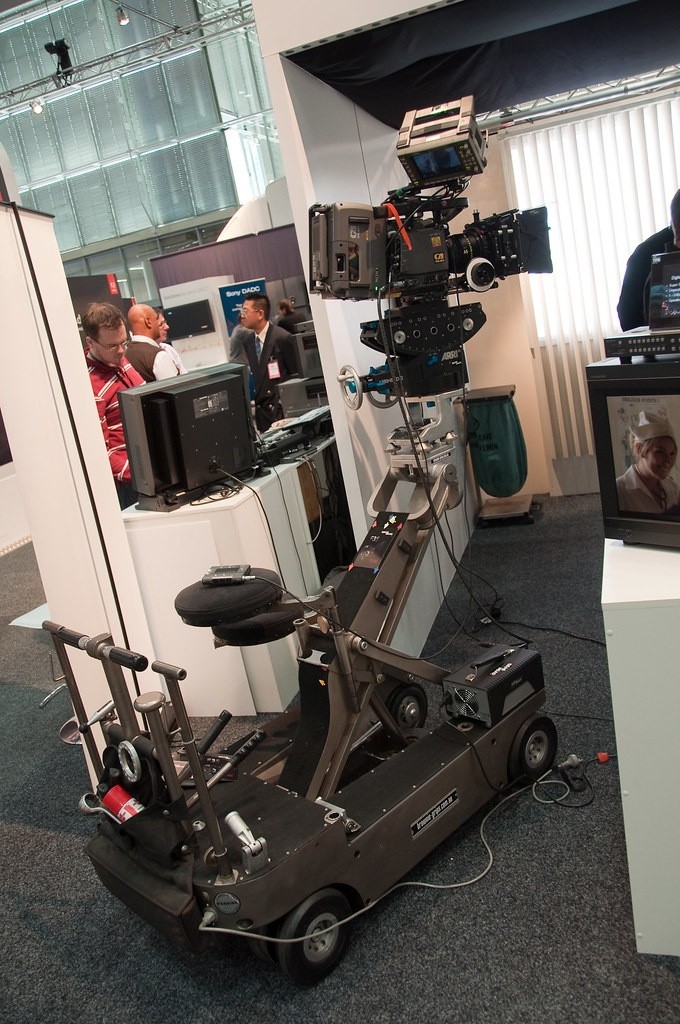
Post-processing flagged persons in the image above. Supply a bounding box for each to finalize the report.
[80,301,147,510]
[151,307,188,375]
[615,409,680,514]
[125,304,180,383]
[227,314,256,365]
[241,293,300,433]
[615,187,680,332]
[272,298,307,335]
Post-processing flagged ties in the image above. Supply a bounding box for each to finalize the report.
[255,336,261,364]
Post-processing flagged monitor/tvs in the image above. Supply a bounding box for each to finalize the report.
[118,364,258,498]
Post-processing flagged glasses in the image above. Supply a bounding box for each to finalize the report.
[241,308,260,314]
[90,333,132,351]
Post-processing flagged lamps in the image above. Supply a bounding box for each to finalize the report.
[115,5,129,25]
[28,100,43,114]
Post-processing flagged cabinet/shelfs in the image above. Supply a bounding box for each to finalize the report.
[599,537,680,956]
[122,435,335,717]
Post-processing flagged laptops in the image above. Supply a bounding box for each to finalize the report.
[649,250,680,331]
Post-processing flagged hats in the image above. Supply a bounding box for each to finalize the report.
[629,409,674,442]
